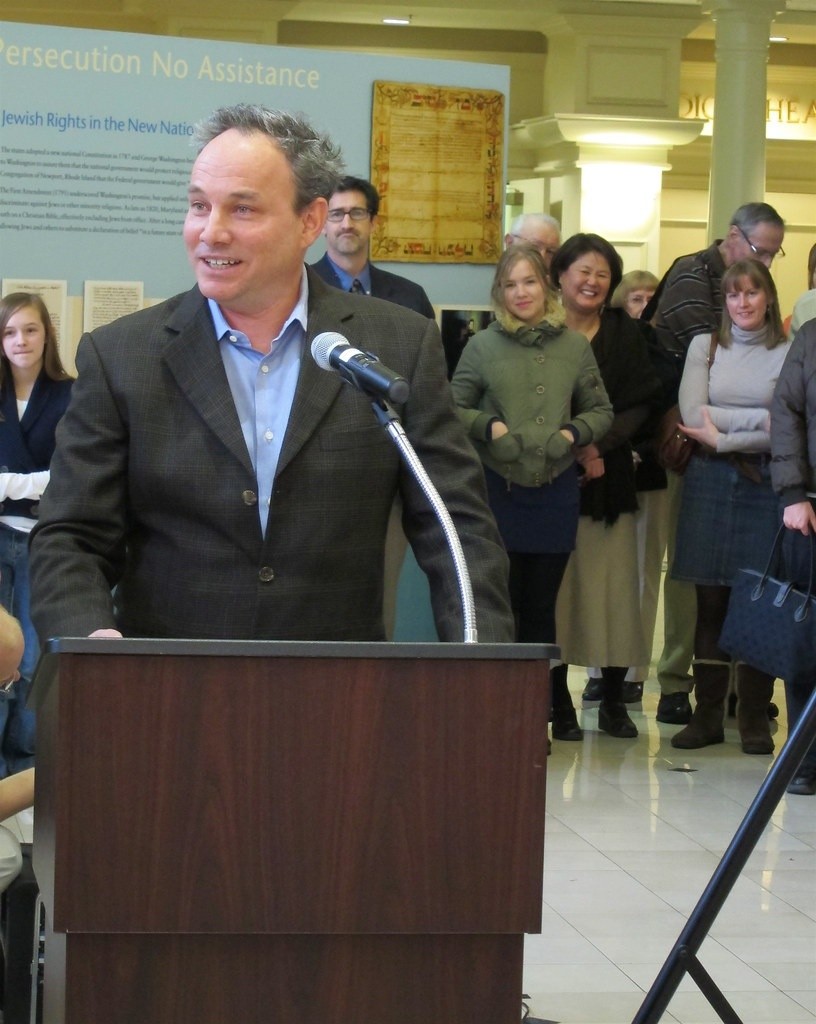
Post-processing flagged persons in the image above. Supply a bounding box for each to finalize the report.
[0,176,816,901]
[28,105,516,642]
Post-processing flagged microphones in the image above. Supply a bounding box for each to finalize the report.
[311,332,412,405]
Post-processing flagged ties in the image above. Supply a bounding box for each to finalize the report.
[351,279,364,296]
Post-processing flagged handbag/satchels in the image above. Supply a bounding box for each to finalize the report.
[715,519,816,686]
[659,329,718,477]
[638,252,712,421]
[577,438,668,494]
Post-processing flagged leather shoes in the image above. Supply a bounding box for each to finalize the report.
[728,692,779,719]
[598,701,638,738]
[656,691,692,725]
[551,703,583,741]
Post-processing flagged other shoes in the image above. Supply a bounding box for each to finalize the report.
[786,754,816,794]
[582,677,605,700]
[617,682,643,702]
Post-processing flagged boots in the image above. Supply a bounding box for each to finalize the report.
[734,661,776,754]
[671,658,730,749]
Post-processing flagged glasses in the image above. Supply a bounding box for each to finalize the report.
[512,231,558,260]
[326,207,373,222]
[730,223,786,258]
